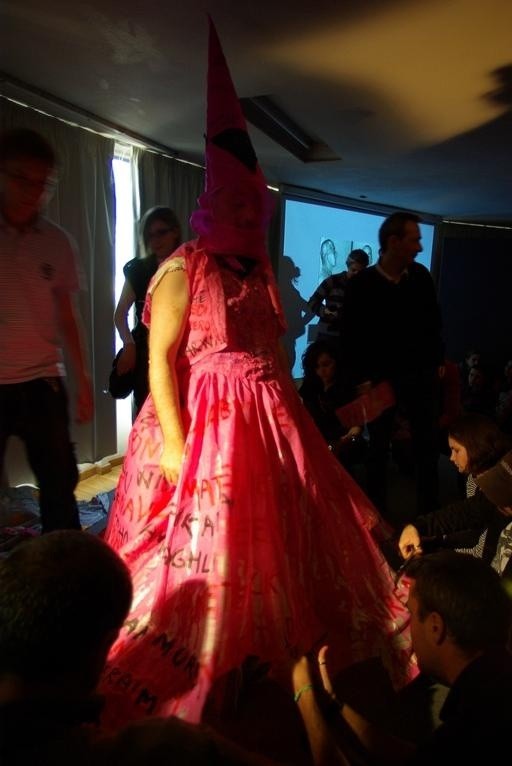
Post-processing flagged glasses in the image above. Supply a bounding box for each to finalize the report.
[146,226,174,241]
[0,166,53,192]
